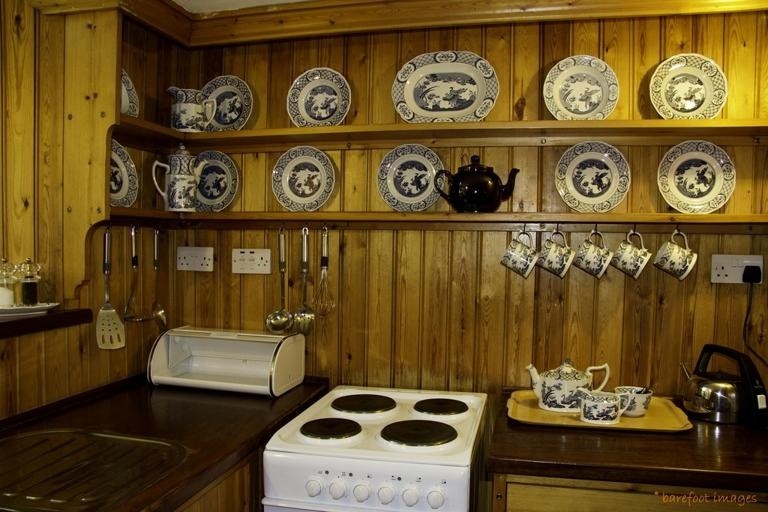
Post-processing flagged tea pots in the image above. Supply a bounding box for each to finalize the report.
[523,362,609,412]
[433,154,520,213]
[151,145,206,212]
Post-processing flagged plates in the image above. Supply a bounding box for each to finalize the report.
[194,67,353,212]
[121,67,140,118]
[389,50,500,124]
[110,138,140,208]
[543,53,738,215]
[0,303,60,317]
[376,143,445,212]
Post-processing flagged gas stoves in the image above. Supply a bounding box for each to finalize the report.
[263,384,488,511]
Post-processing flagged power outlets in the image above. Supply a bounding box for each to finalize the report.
[232,248,271,274]
[177,247,215,271]
[712,254,764,286]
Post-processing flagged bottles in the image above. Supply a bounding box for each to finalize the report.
[1,258,41,307]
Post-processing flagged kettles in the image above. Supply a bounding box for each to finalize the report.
[679,342,768,425]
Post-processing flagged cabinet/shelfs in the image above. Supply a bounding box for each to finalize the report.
[488,469,766,512]
[176,450,260,512]
[38,1,768,224]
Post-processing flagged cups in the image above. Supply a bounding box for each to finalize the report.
[499,226,698,279]
[581,391,632,425]
[170,87,218,133]
[614,385,653,417]
